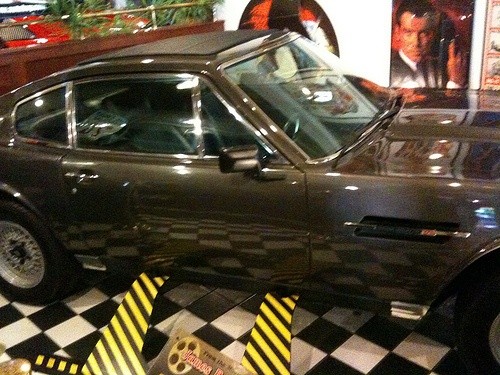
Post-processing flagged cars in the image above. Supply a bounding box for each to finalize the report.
[0,28,500,373]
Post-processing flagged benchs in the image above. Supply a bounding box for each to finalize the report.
[21,86,143,154]
